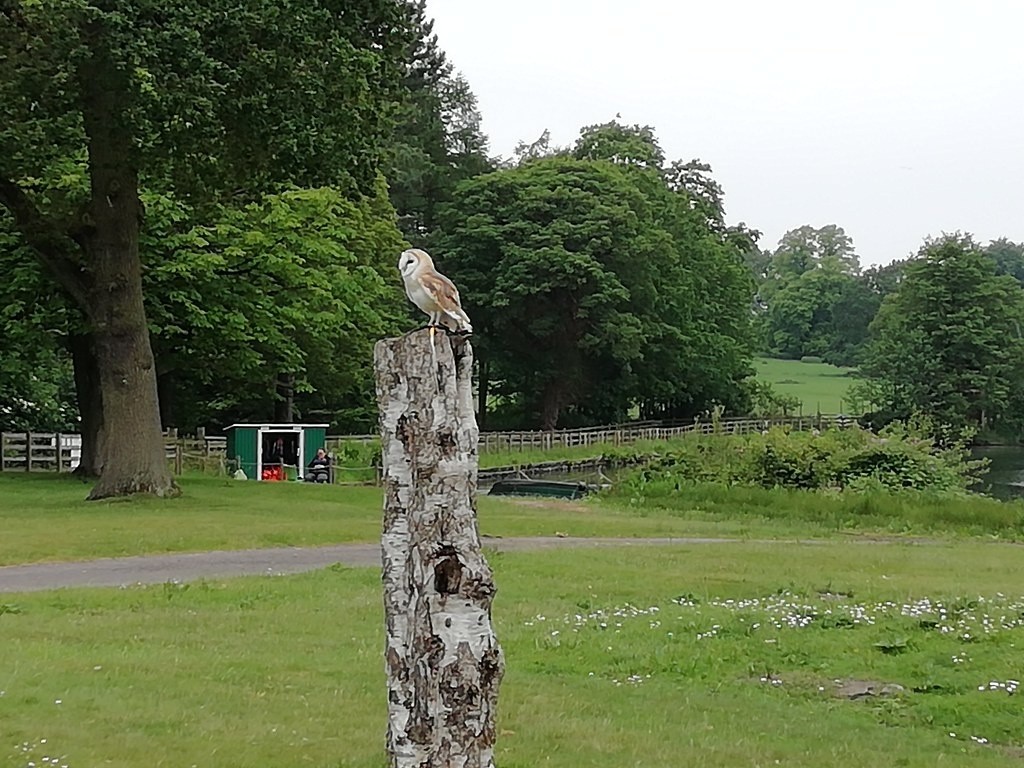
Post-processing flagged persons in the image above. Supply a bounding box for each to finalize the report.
[304,447,330,483]
[272,438,292,464]
